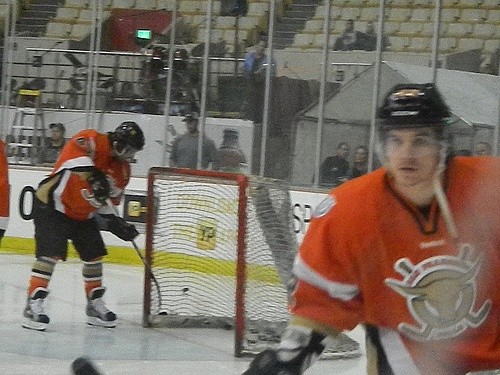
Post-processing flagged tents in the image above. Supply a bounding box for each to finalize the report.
[288,60,500,185]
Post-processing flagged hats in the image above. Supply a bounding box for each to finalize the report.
[182,112,199,122]
[49,122,65,131]
[222,128,239,137]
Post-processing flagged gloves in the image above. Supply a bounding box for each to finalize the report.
[241,350,301,375]
[110,217,139,241]
[89,172,111,202]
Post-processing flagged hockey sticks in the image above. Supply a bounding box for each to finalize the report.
[104,195,168,316]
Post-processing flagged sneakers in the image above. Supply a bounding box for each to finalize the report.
[22,288,50,331]
[85,286,117,328]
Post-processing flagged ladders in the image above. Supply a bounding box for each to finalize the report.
[5,89,47,166]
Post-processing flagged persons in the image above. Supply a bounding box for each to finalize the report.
[242,79,500,375]
[5,19,500,189]
[23,121,146,331]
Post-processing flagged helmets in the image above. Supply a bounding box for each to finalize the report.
[375,83,452,130]
[151,49,162,60]
[115,122,145,152]
[173,48,189,60]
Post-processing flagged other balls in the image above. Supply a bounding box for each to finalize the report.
[183,288,189,292]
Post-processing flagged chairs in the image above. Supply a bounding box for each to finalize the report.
[42,0,500,69]
[0,0,29,36]
